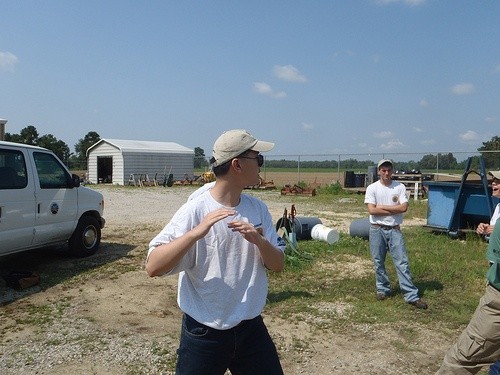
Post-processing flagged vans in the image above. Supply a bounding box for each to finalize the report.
[0,141,105,257]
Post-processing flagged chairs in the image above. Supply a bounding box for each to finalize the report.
[0,167,26,189]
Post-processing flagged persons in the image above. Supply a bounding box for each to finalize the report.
[365,160,428,309]
[433,217,500,375]
[188,181,216,202]
[146,130,285,375]
[476,171,500,239]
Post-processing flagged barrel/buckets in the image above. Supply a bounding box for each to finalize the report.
[344,166,377,187]
[311,224,340,245]
[276,217,323,242]
[350,218,369,239]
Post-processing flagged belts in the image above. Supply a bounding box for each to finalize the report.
[372,224,400,230]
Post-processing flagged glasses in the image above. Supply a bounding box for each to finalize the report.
[238,155,263,167]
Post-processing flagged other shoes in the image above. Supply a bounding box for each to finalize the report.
[409,299,428,309]
[376,293,386,300]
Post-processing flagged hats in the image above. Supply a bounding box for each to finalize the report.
[378,159,394,170]
[213,130,275,168]
[489,171,500,179]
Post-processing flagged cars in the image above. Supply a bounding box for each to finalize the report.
[393,169,419,174]
